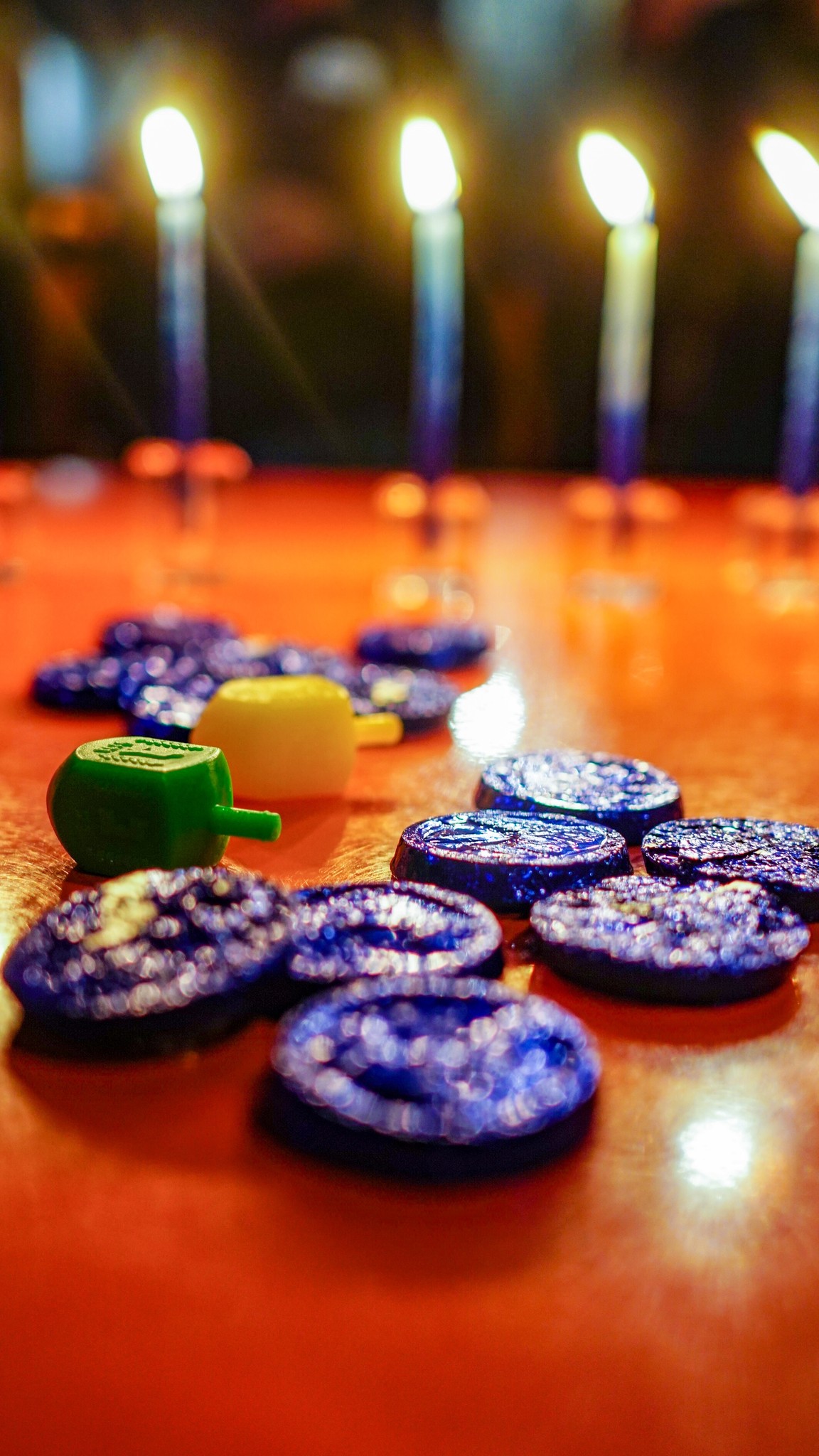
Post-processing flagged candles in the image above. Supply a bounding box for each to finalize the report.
[597,203,659,485]
[780,229,819,500]
[158,202,208,441]
[412,204,464,481]
